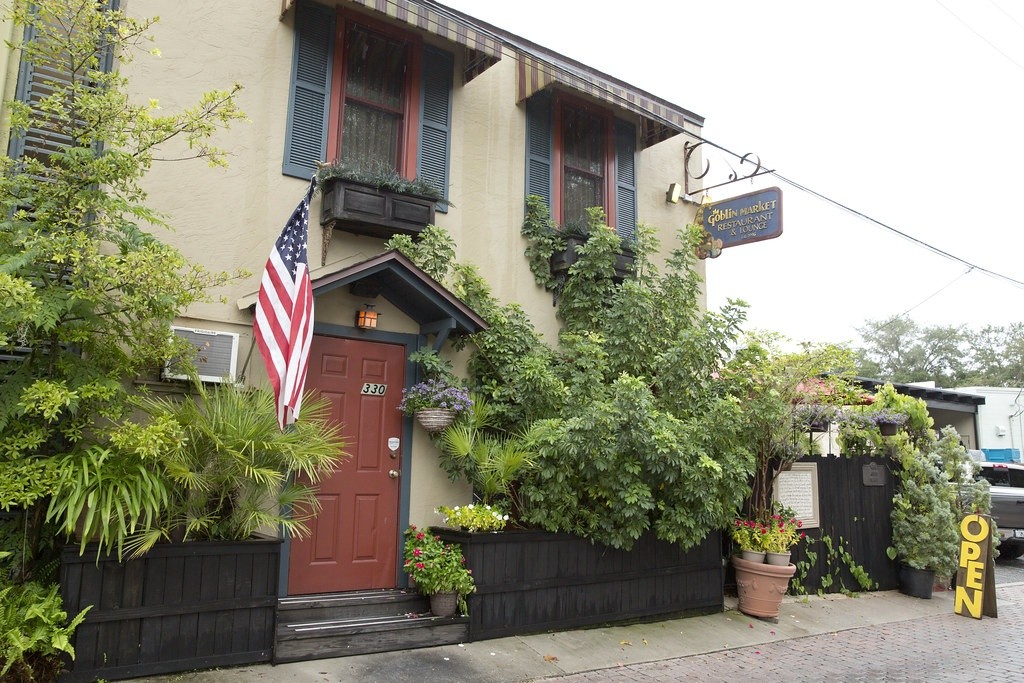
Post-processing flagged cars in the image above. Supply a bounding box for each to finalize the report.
[933,461,1023,529]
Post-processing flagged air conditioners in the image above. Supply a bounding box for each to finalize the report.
[160,325,241,387]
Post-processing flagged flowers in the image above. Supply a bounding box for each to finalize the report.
[764,511,804,554]
[397,372,476,416]
[869,408,903,429]
[402,523,477,617]
[733,515,768,552]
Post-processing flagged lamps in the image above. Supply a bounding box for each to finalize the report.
[355,302,382,330]
[666,183,682,204]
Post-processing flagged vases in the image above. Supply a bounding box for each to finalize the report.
[765,552,794,567]
[416,409,456,433]
[740,549,766,562]
[880,423,897,436]
[732,554,796,618]
[430,591,457,617]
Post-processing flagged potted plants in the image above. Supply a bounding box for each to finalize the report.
[319,152,440,241]
[550,215,637,280]
[890,455,963,601]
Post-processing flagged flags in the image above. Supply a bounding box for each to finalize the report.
[254,195,314,429]
